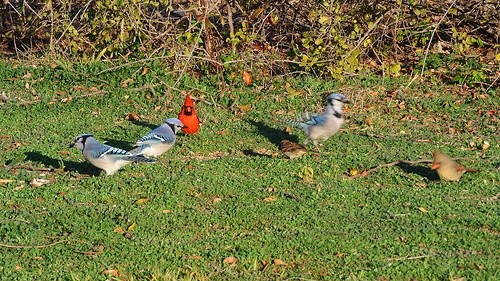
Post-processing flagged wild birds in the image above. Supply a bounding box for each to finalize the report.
[126,117,188,158]
[277,93,352,153]
[68,134,162,178]
[429,148,479,182]
[239,69,252,86]
[181,93,200,135]
